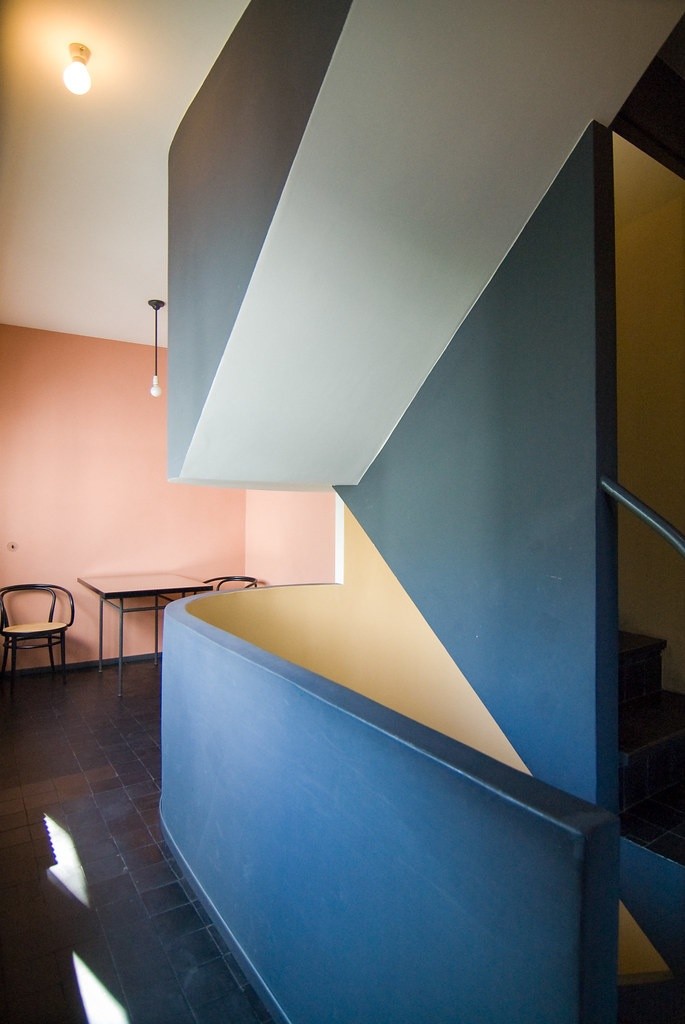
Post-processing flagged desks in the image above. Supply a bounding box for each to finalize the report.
[77,574,215,698]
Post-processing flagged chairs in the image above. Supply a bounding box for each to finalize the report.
[195,577,260,600]
[0,584,74,695]
